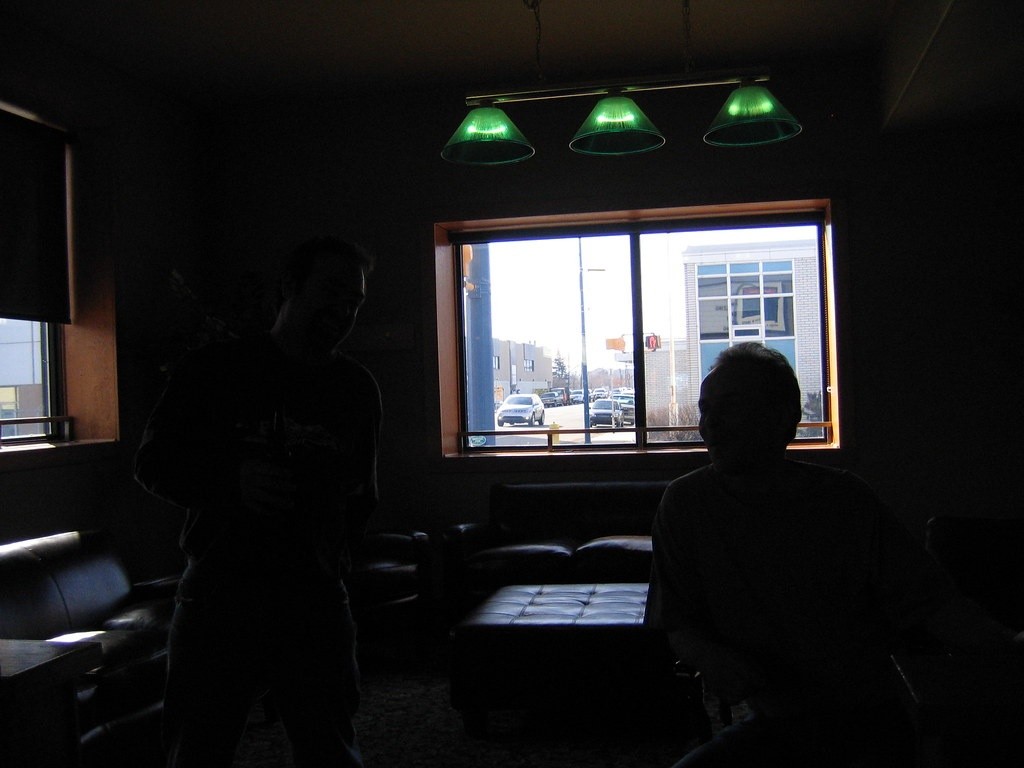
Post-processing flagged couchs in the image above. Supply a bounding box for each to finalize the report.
[924,514,1024,631]
[337,529,437,677]
[0,526,183,768]
[441,481,669,645]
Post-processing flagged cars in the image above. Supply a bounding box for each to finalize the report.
[540,392,563,408]
[496,394,545,426]
[551,388,635,425]
[589,399,624,427]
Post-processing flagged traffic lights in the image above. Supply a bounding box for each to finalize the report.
[646,336,660,349]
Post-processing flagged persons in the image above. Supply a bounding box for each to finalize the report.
[132,234,383,768]
[639,356,1024,768]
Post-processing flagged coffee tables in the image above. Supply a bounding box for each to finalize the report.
[448,582,707,738]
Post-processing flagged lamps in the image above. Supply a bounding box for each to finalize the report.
[701,79,803,147]
[440,102,534,166]
[568,89,666,157]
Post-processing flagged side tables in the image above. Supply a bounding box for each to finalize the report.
[885,653,1024,768]
